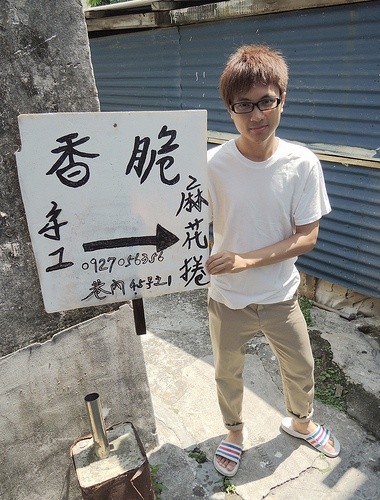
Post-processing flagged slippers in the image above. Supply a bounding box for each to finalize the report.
[281,416,341,458]
[213,439,243,478]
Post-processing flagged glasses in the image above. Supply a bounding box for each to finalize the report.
[229,95,281,114]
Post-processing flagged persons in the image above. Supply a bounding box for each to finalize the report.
[208,44,342,478]
